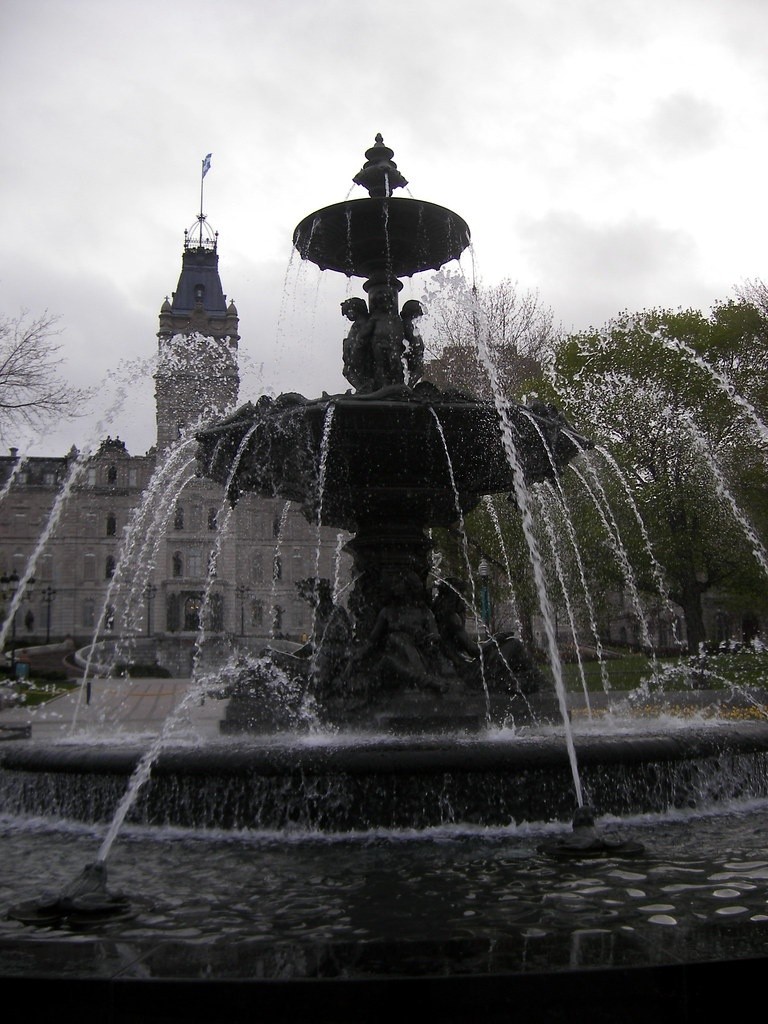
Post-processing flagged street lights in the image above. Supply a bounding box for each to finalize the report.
[0,568,36,679]
[478,558,492,637]
[41,585,57,645]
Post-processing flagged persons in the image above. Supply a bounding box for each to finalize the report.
[290,576,542,704]
[339,297,371,392]
[301,633,307,644]
[400,299,427,388]
